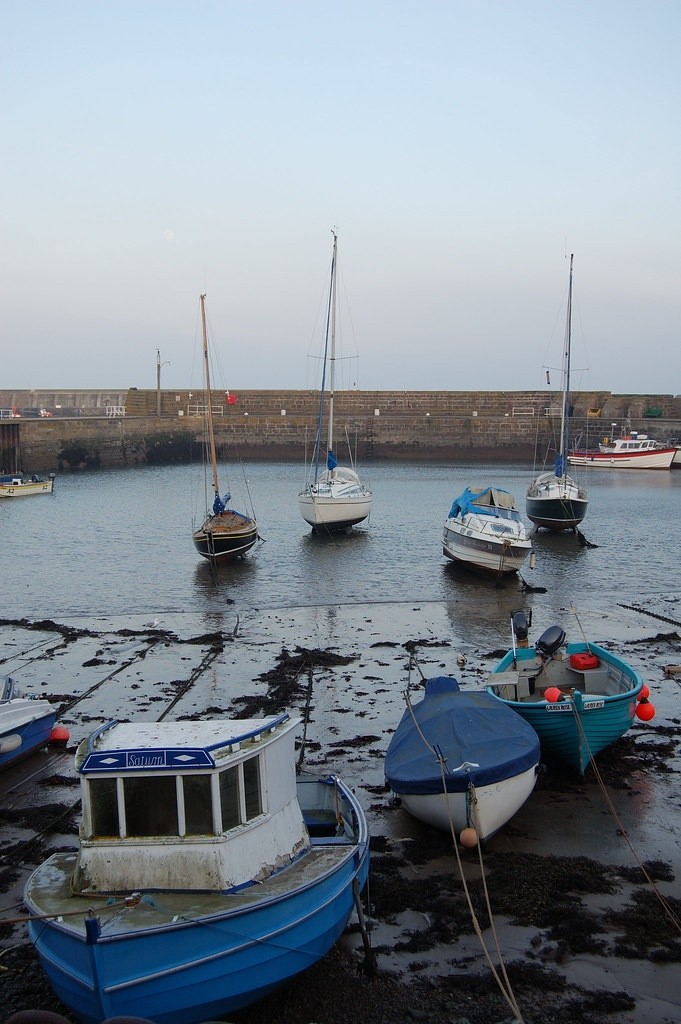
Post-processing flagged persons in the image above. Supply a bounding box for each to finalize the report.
[160,787,212,834]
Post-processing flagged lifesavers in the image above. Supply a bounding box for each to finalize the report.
[32,473,39,482]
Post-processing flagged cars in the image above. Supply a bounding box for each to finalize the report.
[38,412,52,418]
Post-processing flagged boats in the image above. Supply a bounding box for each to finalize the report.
[440,484,534,578]
[0,676,58,771]
[25,711,374,1023]
[485,606,645,784]
[568,436,678,471]
[0,472,56,498]
[655,441,681,470]
[381,672,543,849]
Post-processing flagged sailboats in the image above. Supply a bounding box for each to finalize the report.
[191,292,261,564]
[298,229,375,533]
[525,254,588,529]
